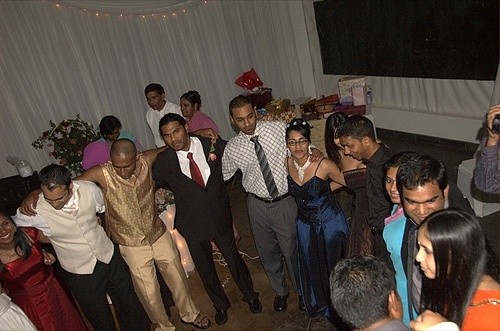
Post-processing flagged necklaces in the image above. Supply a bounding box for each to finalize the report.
[338,116,394,271]
[293,155,313,182]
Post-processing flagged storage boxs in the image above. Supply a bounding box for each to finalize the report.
[341,105,368,117]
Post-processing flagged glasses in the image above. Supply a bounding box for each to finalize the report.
[288,136,309,146]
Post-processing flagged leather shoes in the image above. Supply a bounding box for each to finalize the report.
[298,295,307,311]
[274,292,289,312]
[247,294,262,313]
[214,304,230,324]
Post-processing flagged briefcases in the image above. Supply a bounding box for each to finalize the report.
[180,313,211,329]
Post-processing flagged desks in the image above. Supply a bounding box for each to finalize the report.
[302,101,375,158]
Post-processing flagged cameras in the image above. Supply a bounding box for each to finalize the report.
[492,114,500,134]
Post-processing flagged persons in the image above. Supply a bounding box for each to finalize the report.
[325,112,390,258]
[19,127,219,331]
[0,285,39,331]
[96,116,144,158]
[180,90,220,138]
[382,151,418,328]
[416,207,500,330]
[144,83,188,149]
[9,163,153,331]
[0,210,102,331]
[328,256,461,331]
[222,96,325,315]
[470,103,500,203]
[151,112,263,324]
[285,117,345,318]
[396,152,450,320]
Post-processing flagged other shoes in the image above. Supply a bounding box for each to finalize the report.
[235,233,242,246]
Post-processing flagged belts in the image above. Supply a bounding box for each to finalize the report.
[250,193,289,204]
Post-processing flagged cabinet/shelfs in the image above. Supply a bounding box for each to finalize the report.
[0,171,42,215]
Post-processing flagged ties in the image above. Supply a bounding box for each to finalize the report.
[187,153,205,188]
[250,136,279,199]
[62,196,77,210]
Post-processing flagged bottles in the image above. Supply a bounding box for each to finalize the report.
[17,160,34,178]
[295,105,302,119]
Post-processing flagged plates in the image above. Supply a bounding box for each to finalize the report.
[318,105,334,114]
